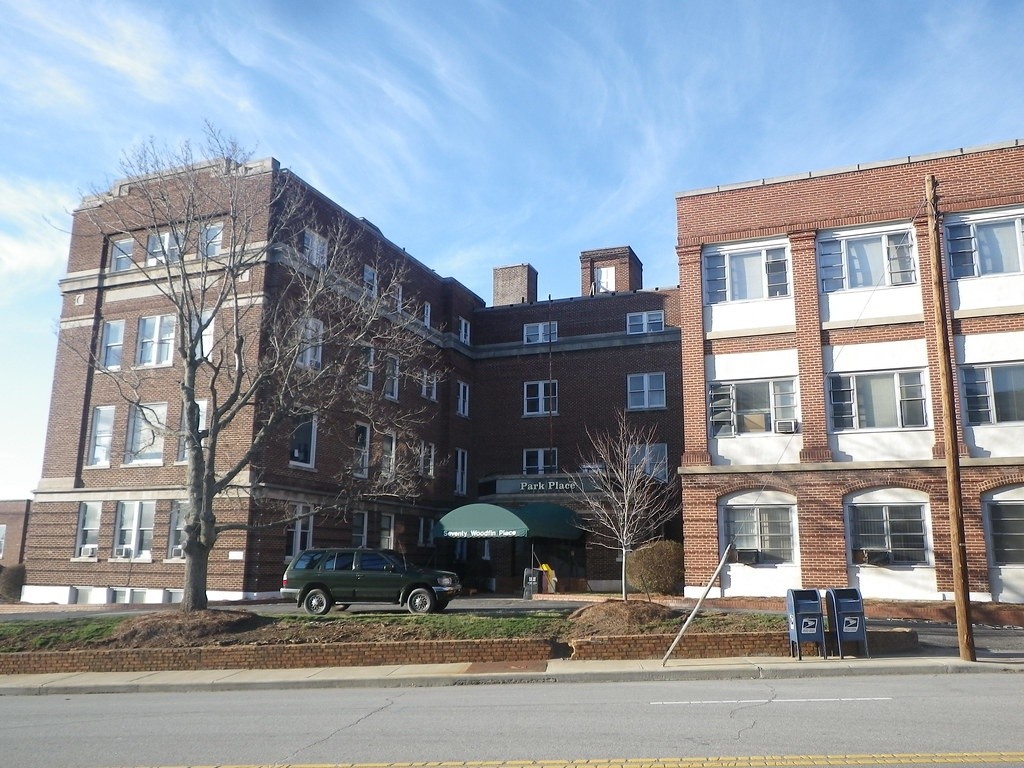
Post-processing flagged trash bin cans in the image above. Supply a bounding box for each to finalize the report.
[534,568,548,594]
[541,564,551,584]
[485,577,496,593]
[787,588,828,662]
[523,568,543,595]
[548,569,556,594]
[576,578,587,593]
[825,586,870,660]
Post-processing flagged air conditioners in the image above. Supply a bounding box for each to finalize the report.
[80,547,96,557]
[172,548,185,559]
[309,359,320,371]
[114,548,129,558]
[775,420,794,433]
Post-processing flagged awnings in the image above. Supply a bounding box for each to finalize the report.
[432,502,582,538]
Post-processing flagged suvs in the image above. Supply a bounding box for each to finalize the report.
[279,547,463,617]
